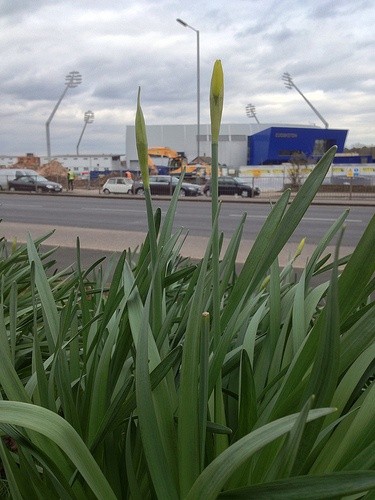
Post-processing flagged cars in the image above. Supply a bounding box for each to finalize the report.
[103,176,134,194]
[7,175,63,193]
[202,176,261,198]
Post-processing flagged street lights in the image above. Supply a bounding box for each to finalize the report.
[76,111,95,155]
[245,103,259,125]
[283,71,329,129]
[45,70,84,162]
[175,17,201,175]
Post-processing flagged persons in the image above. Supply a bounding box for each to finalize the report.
[124,169,132,180]
[67,168,74,191]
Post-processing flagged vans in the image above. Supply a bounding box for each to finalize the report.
[0,168,49,190]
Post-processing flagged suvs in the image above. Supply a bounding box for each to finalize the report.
[131,174,202,197]
